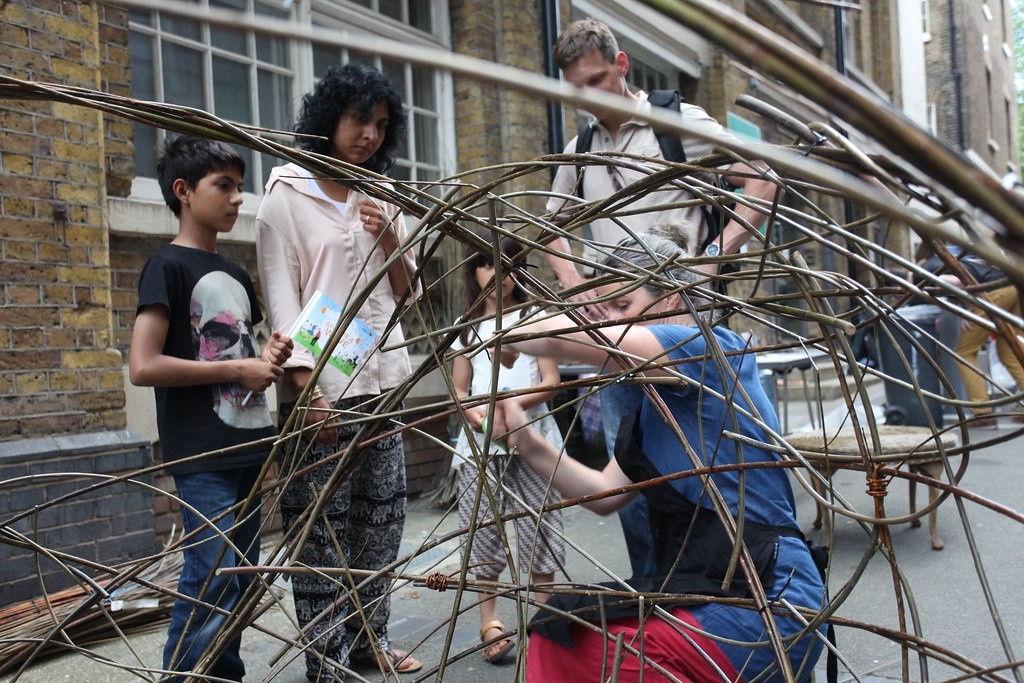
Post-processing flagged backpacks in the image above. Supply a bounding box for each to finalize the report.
[574,89,742,285]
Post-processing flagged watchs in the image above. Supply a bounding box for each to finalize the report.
[705,242,728,265]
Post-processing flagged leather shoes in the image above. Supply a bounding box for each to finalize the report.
[965,413,999,431]
[1012,412,1024,425]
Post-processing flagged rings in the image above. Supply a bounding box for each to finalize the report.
[366,215,370,224]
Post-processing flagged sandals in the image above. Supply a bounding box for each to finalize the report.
[358,647,422,673]
[480,620,515,662]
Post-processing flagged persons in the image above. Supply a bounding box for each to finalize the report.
[442,229,569,663]
[257,65,424,683]
[880,259,1024,430]
[540,16,777,579]
[483,216,838,683]
[130,134,293,683]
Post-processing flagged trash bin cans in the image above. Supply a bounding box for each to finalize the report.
[844,304,949,431]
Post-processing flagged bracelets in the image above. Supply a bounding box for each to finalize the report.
[296,385,324,406]
